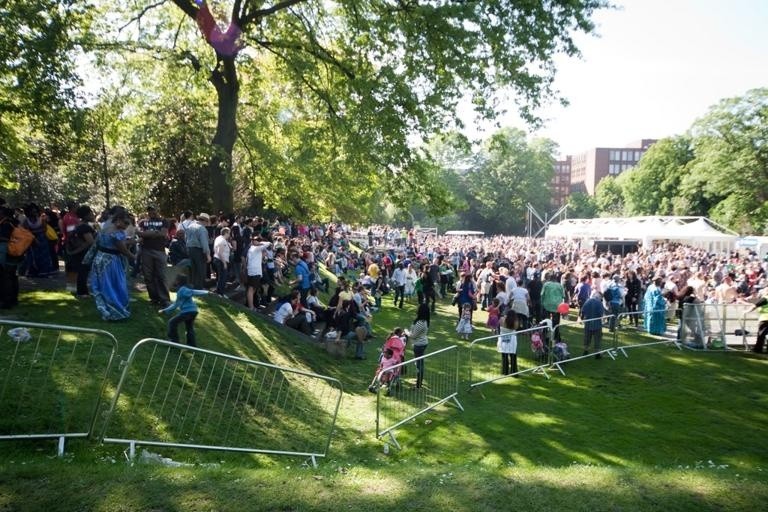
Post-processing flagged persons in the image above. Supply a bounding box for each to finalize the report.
[157,212,767,378]
[376,302,429,392]
[0,199,170,320]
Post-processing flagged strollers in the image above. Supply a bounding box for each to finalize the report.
[527,320,569,366]
[368,332,409,395]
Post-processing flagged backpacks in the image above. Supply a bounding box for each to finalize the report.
[604,285,619,302]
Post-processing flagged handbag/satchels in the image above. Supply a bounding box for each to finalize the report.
[45,224,58,241]
[8,222,35,256]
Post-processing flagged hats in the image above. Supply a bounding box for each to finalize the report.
[196,213,210,221]
[613,275,623,281]
[146,205,156,210]
